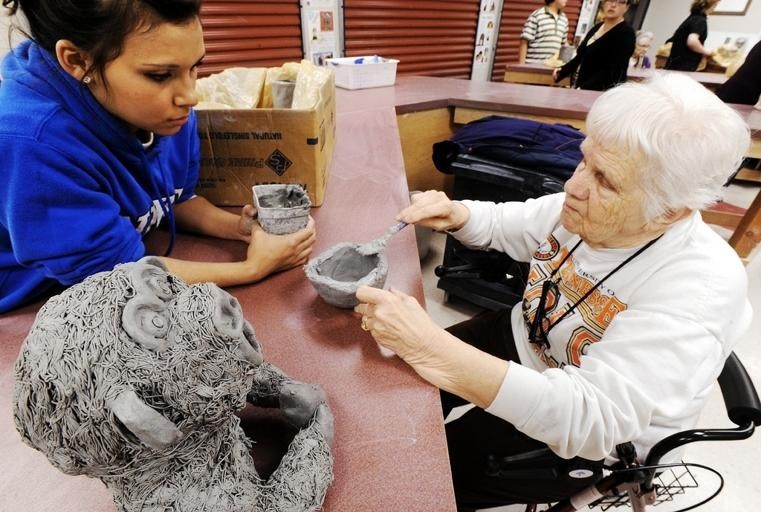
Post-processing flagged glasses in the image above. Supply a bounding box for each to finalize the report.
[527,279,564,351]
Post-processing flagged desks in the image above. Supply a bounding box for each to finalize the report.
[2,65,761,512]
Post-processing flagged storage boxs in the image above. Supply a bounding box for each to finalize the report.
[326,52,399,91]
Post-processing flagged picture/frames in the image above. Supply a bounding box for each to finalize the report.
[711,0,752,16]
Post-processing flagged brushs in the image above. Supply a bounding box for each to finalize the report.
[356,222,409,256]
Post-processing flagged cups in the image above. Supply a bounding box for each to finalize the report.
[248,181,315,236]
[303,239,391,310]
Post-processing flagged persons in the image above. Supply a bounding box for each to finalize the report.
[518,1,572,67]
[1,0,319,321]
[344,69,759,511]
[551,0,639,92]
[662,1,719,74]
